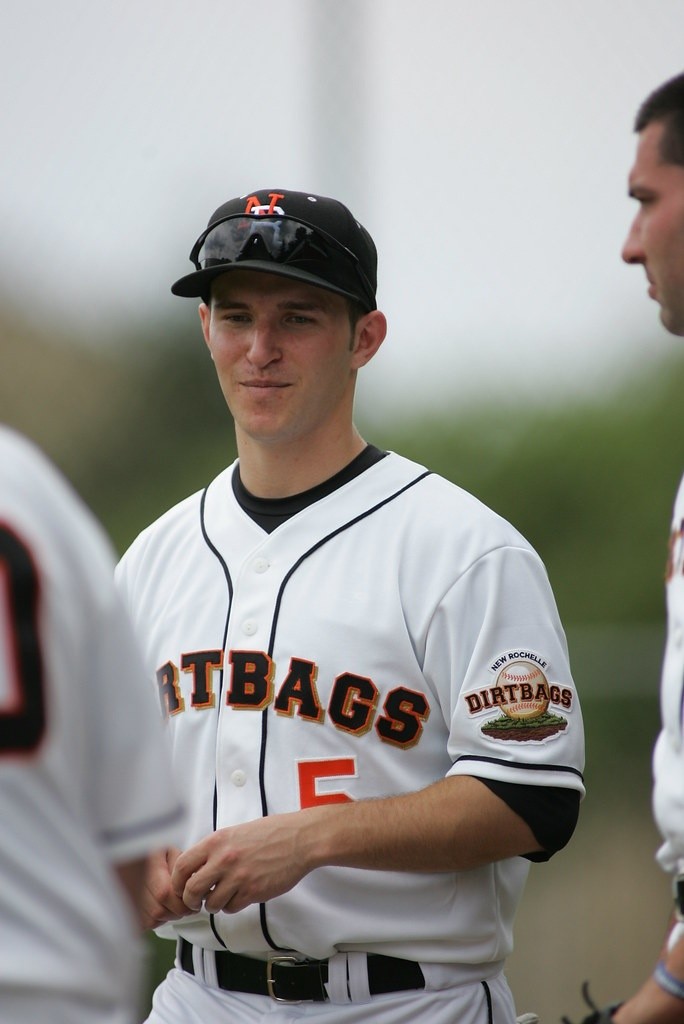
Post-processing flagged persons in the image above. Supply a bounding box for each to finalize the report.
[111,190,583,1024]
[0,421,185,1024]
[564,72,684,1024]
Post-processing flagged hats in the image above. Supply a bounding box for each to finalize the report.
[171,188,379,312]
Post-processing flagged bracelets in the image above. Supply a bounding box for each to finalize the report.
[653,962,684,999]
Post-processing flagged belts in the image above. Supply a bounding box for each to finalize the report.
[180,937,425,1003]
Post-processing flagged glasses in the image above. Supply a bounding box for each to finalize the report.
[190,213,374,298]
